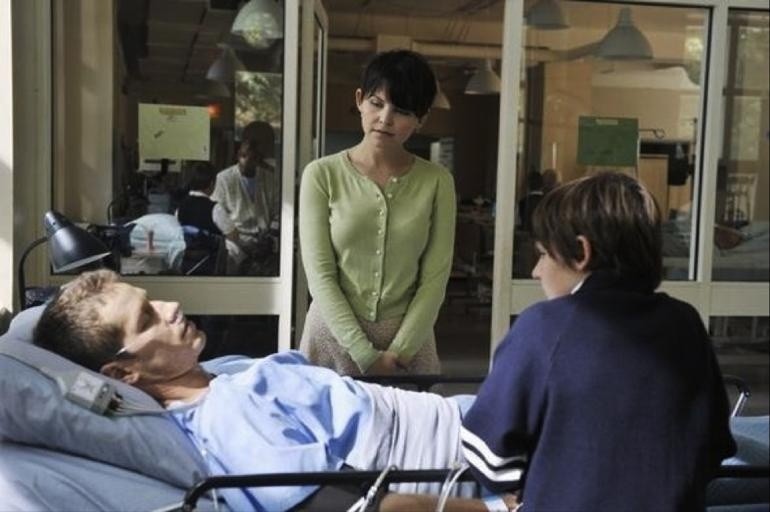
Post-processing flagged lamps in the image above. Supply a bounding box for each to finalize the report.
[464,58,502,96]
[593,2,656,62]
[19,210,112,311]
[205,3,284,84]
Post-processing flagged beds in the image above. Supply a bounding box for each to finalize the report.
[0,304,770,511]
[472,214,770,344]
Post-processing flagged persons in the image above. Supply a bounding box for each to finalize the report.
[520,171,746,255]
[296,47,458,392]
[111,122,280,278]
[34,269,518,512]
[460,169,738,512]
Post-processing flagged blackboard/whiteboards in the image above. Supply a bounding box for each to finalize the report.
[575,114,640,168]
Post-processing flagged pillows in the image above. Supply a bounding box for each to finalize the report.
[2,306,227,501]
